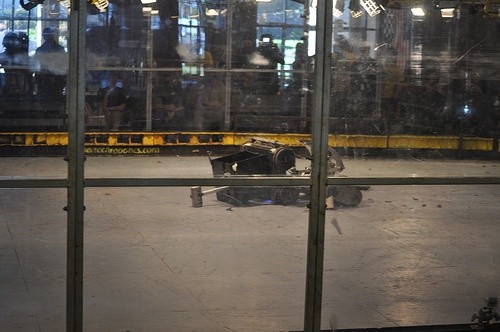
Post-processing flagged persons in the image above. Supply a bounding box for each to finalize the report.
[104,78,134,131]
[192,64,228,143]
[329,33,399,127]
[0,32,35,93]
[34,24,67,96]
